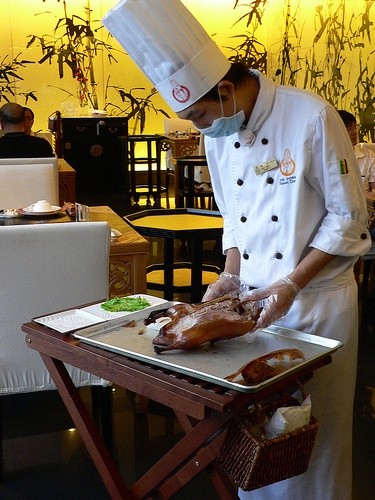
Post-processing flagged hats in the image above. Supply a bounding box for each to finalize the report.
[100,0,232,112]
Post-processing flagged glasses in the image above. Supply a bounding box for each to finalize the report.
[348,123,359,133]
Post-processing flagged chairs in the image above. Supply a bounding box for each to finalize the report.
[163,118,211,182]
[0,131,60,209]
[0,221,116,418]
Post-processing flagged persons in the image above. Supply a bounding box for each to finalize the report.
[22,107,51,144]
[102,0,372,499]
[0,103,54,158]
[336,111,375,192]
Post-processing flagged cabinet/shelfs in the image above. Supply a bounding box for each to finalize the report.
[174,156,216,212]
[124,205,226,304]
[125,132,166,207]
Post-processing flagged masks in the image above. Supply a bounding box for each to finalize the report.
[191,82,245,139]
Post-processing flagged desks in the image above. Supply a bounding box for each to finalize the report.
[23,296,338,499]
[1,206,149,296]
[58,159,79,206]
[156,131,200,157]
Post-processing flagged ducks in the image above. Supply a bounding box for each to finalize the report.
[144,294,263,354]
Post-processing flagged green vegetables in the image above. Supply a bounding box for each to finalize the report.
[100,297,150,312]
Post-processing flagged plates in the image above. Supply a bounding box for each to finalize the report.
[15,205,61,215]
